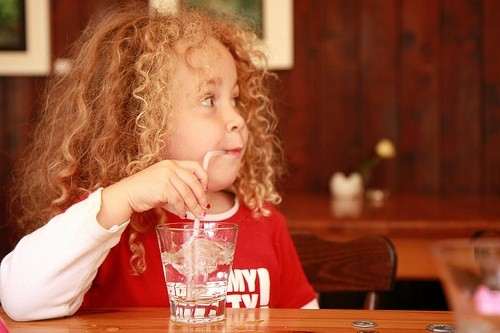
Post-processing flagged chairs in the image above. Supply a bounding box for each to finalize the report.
[291,234,397,309]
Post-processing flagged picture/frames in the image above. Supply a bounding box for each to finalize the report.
[148,0,294,69]
[0,0,50,77]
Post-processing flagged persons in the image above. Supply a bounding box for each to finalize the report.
[0,3,321,322]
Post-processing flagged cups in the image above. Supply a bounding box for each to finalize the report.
[155,222,239,324]
[431,238,500,333]
[362,162,391,207]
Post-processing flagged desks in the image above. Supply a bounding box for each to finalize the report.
[0,308,455,333]
[280,196,500,282]
